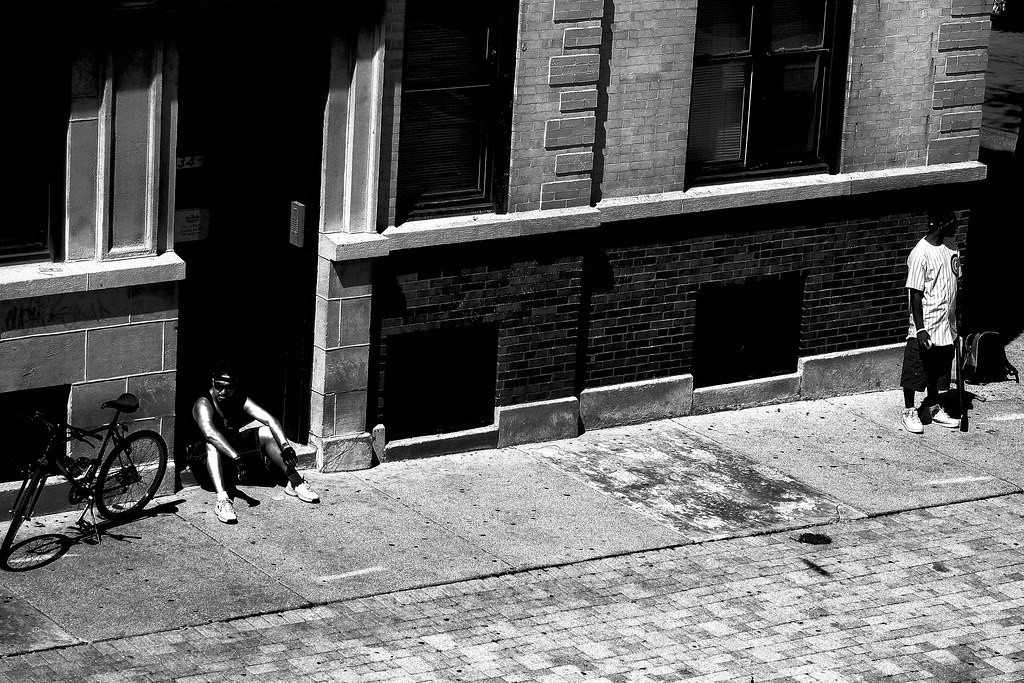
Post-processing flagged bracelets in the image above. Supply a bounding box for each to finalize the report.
[916,329,925,334]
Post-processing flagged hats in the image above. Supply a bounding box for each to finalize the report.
[211,361,237,383]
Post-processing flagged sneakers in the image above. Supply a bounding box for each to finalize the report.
[215,497,237,523]
[285,476,319,503]
[902,407,923,432]
[922,403,960,428]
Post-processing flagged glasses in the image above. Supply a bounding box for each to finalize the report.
[213,381,234,391]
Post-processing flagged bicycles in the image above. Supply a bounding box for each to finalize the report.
[0,389,169,567]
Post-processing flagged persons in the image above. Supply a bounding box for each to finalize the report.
[901,208,962,433]
[188,369,319,524]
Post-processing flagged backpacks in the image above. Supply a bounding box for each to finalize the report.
[961,329,1019,382]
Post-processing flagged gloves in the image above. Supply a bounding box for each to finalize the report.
[233,456,247,481]
[280,446,298,470]
